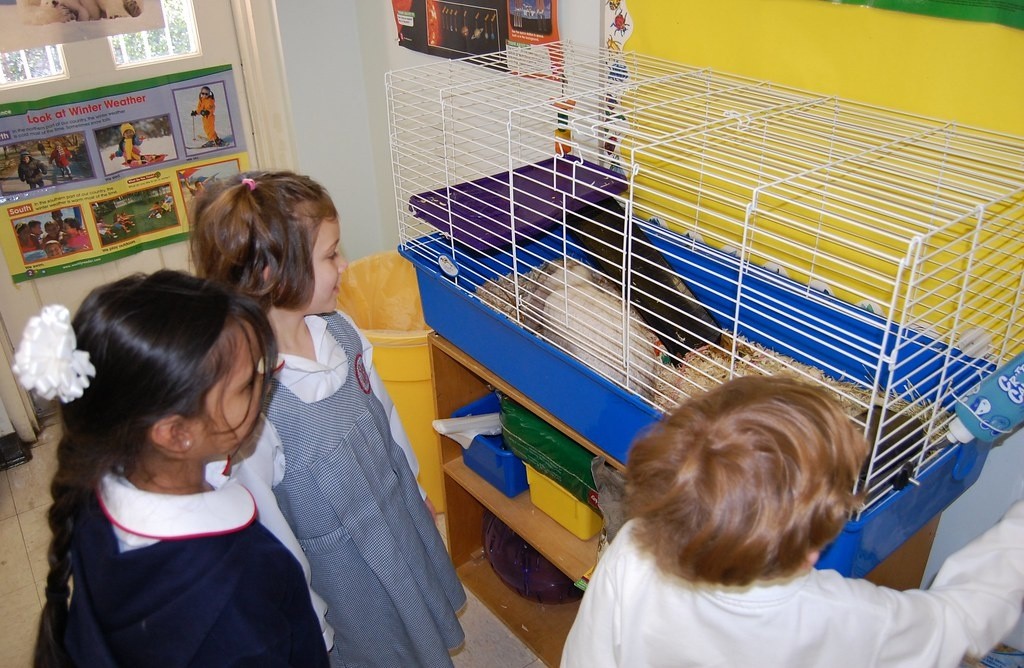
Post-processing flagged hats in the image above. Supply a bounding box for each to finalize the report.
[121,123,135,137]
[21,151,30,156]
[55,141,61,146]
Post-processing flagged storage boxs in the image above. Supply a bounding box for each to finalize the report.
[450,390,529,498]
[397,213,999,580]
[523,461,606,541]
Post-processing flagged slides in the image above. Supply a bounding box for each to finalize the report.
[572,198,723,368]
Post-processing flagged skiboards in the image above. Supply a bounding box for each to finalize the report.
[185,133,232,150]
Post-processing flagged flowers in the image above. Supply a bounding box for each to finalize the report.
[12,303,96,404]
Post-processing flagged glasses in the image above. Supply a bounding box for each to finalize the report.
[202,92,208,95]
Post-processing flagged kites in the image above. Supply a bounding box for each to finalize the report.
[178,168,199,178]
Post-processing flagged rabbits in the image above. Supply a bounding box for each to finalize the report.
[525,260,654,404]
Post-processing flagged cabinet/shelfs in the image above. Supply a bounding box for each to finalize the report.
[427,331,941,668]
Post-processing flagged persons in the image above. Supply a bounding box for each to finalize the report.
[17,140,75,190]
[184,170,220,201]
[108,121,161,168]
[190,86,225,148]
[55,1,101,22]
[556,374,1024,668]
[188,171,469,668]
[96,192,175,239]
[31,266,334,668]
[13,210,91,259]
